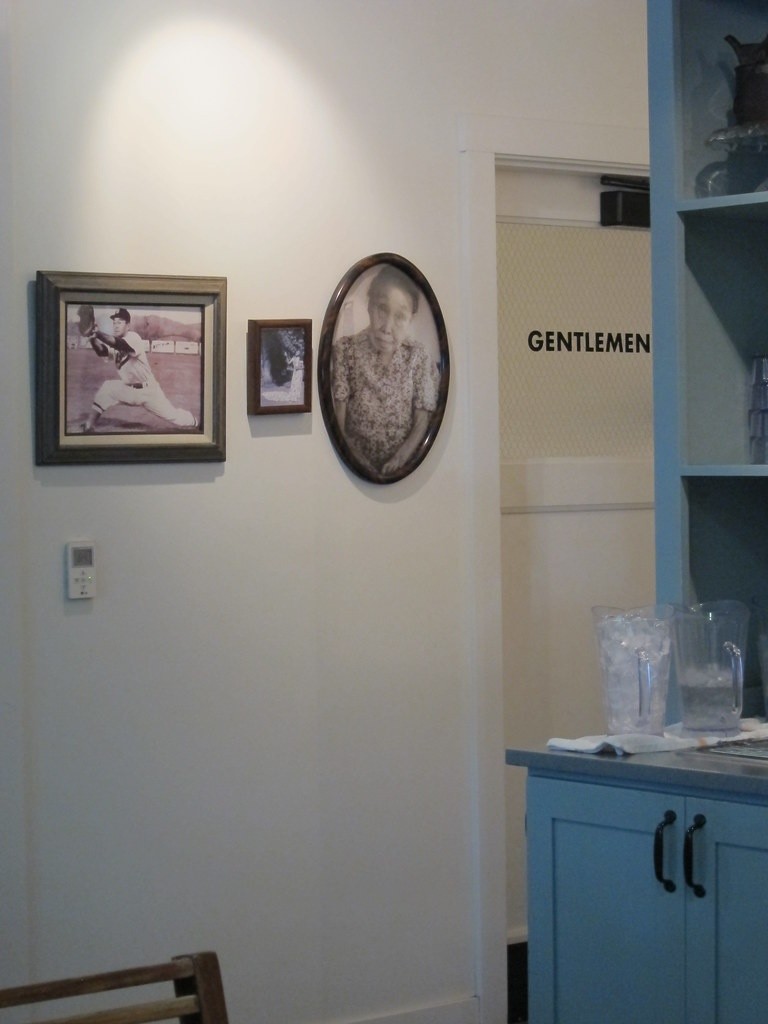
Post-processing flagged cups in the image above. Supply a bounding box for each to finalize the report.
[675,598,750,738]
[589,603,674,740]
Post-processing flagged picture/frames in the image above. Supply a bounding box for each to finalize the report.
[317,252,450,486]
[35,270,228,466]
[247,318,312,416]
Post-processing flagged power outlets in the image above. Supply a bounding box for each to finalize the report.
[67,540,97,600]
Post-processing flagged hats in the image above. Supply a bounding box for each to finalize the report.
[110,308,131,324]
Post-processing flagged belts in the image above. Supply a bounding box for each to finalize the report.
[125,383,149,388]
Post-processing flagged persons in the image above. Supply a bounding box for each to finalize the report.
[329,261,439,481]
[283,348,304,404]
[74,305,204,433]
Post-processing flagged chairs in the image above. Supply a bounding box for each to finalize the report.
[0,951,231,1024]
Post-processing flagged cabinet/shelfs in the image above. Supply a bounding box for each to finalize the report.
[648,2,768,738]
[504,729,768,1023]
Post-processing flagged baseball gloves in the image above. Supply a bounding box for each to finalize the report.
[76,305,96,336]
